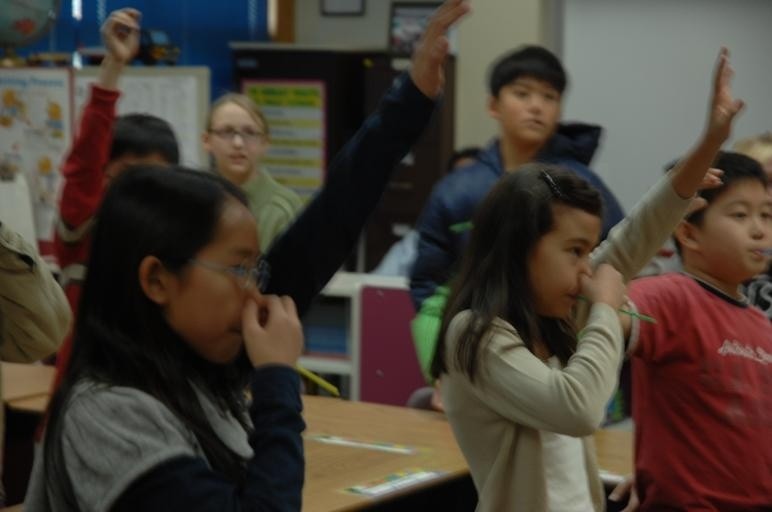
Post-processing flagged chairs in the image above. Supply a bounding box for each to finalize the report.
[348,274,430,409]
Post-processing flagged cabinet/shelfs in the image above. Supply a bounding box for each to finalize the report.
[229,40,457,404]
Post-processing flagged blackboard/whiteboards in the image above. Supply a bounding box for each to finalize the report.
[549,0,772,275]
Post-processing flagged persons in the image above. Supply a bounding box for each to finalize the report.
[34,8,180,452]
[429,47,745,512]
[408,44,626,316]
[605,152,772,512]
[202,93,303,255]
[732,131,772,320]
[0,221,73,365]
[23,0,472,511]
[373,147,480,277]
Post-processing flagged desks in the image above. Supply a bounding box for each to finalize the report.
[298,393,470,512]
[589,417,638,485]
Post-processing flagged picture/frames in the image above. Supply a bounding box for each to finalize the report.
[320,1,367,16]
[387,0,450,53]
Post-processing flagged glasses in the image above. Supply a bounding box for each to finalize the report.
[209,126,264,141]
[193,258,268,290]
